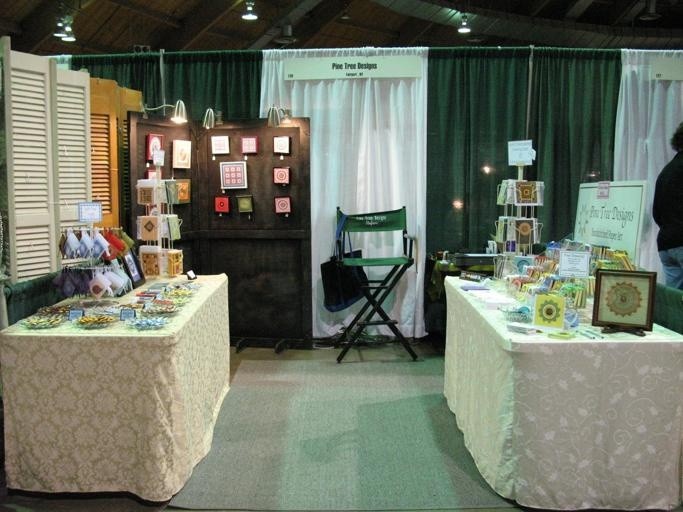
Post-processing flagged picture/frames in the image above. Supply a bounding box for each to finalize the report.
[142,132,292,218]
[589,268,660,333]
[120,247,147,291]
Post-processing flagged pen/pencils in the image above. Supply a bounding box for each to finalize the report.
[576,329,595,339]
[587,330,604,339]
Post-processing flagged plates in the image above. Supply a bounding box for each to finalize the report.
[21,316,62,329]
[73,316,112,328]
[126,316,171,330]
[141,281,200,315]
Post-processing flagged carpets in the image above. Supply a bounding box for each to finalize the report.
[166,352,517,512]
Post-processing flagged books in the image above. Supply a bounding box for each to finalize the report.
[488,179,633,330]
[137,178,185,278]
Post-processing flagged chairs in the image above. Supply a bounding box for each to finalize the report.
[332,205,421,365]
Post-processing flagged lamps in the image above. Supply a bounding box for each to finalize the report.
[240,1,260,23]
[453,13,476,34]
[264,103,294,129]
[51,13,79,46]
[198,106,223,130]
[138,100,188,124]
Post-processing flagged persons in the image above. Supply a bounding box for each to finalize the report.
[652,123,683,294]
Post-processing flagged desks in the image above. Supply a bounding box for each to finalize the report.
[437,271,683,512]
[0,265,234,510]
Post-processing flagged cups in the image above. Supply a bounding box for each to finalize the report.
[88,268,129,299]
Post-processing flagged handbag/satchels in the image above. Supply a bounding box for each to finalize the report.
[320,216,370,312]
